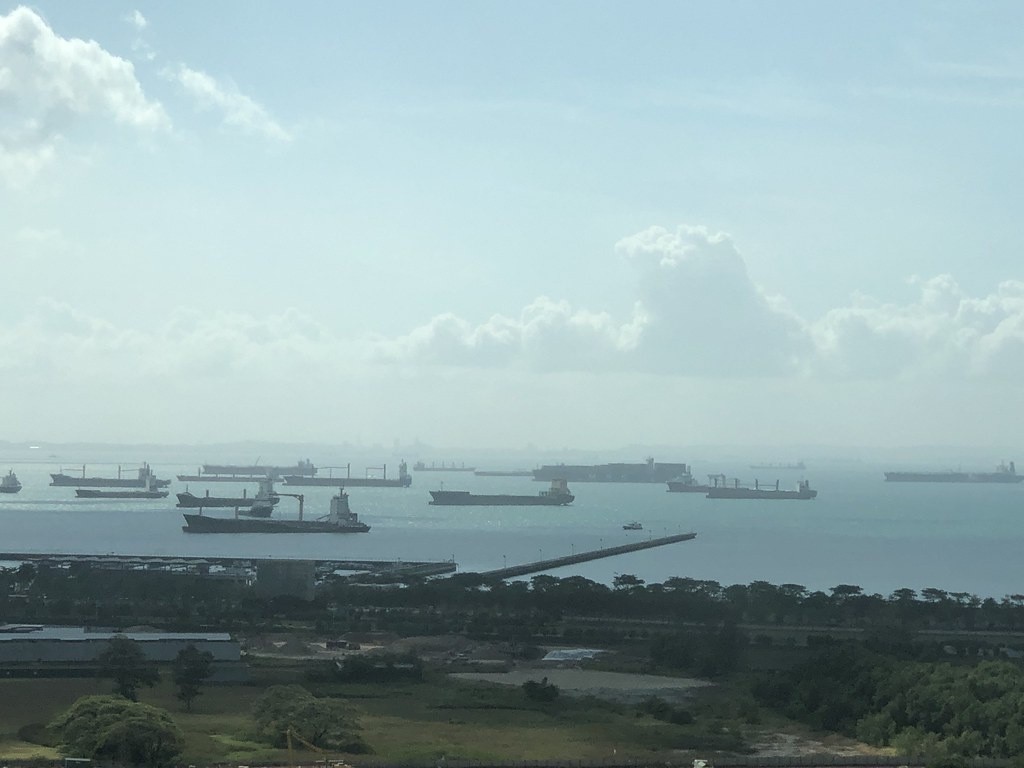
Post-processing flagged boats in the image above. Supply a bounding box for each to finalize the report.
[623,521,644,529]
[0,469,23,494]
[532,457,695,483]
[883,471,1023,483]
[414,460,478,472]
[75,469,172,498]
[48,471,173,488]
[428,476,575,507]
[282,458,413,488]
[706,482,819,500]
[177,469,286,484]
[666,472,751,493]
[201,462,317,476]
[176,482,282,506]
[181,484,373,534]
